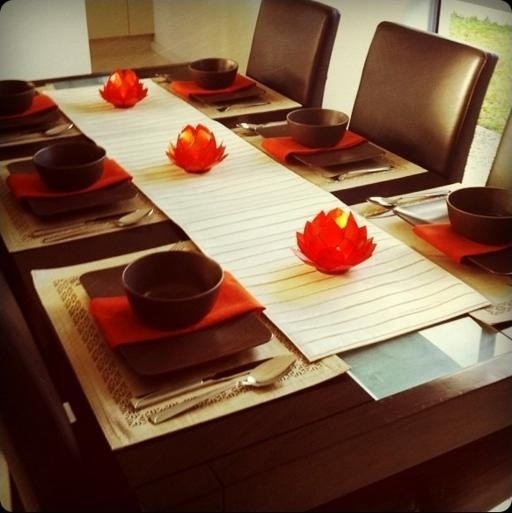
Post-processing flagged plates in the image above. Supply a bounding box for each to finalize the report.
[446,185,511,244]
[392,193,511,275]
[1,90,59,128]
[163,63,265,103]
[80,261,273,381]
[253,123,386,168]
[7,158,137,218]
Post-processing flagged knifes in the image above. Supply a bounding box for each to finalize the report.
[131,356,274,410]
[29,207,133,237]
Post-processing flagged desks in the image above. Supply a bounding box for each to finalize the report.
[0,63,512,512]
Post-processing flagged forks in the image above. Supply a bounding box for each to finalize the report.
[318,164,389,182]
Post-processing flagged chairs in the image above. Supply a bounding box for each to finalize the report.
[246,0,340,108]
[348,21,498,185]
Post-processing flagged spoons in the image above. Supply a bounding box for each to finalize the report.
[235,119,289,131]
[367,189,450,209]
[150,352,296,425]
[1,120,73,145]
[43,206,153,243]
[217,97,272,112]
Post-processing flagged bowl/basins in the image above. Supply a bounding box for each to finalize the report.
[1,80,35,112]
[33,141,107,191]
[286,106,348,146]
[120,247,225,330]
[187,57,238,88]
